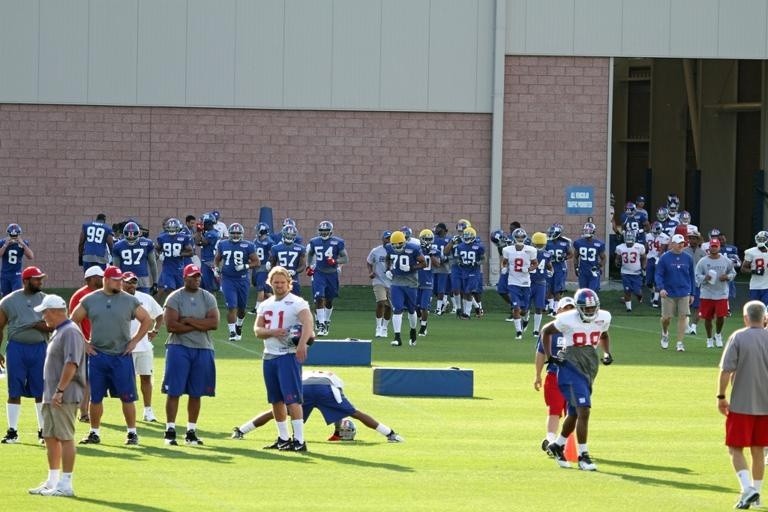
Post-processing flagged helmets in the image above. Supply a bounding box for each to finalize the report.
[622,194,722,248]
[279,323,316,350]
[7,223,25,234]
[491,223,596,246]
[382,219,476,251]
[574,287,601,323]
[125,211,336,240]
[755,231,768,249]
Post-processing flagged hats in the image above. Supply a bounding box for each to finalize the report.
[122,271,138,281]
[104,266,126,281]
[183,264,202,277]
[22,266,45,280]
[557,296,576,308]
[84,265,105,279]
[33,294,67,312]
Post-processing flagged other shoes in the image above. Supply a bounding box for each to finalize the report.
[78,415,89,422]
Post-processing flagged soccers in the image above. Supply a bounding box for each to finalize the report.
[340,419,356,440]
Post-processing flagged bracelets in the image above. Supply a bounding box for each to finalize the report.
[717,394,725,399]
[2,244,7,249]
[152,329,159,334]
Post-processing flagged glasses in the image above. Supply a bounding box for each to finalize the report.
[126,281,137,284]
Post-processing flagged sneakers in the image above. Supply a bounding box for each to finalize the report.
[235,324,243,340]
[736,487,760,509]
[541,439,553,456]
[374,303,540,346]
[79,431,100,445]
[40,488,74,496]
[125,432,138,446]
[319,322,333,339]
[142,406,153,422]
[28,481,50,494]
[577,451,597,470]
[263,436,291,449]
[229,332,236,341]
[184,429,204,446]
[247,308,256,315]
[227,427,244,440]
[38,428,46,446]
[553,312,557,316]
[659,323,725,350]
[279,438,306,451]
[753,496,760,505]
[1,428,19,444]
[163,427,178,447]
[387,429,402,443]
[547,443,571,468]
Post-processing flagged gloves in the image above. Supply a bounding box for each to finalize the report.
[212,256,335,277]
[542,354,566,366]
[600,352,613,365]
[500,266,557,276]
[328,435,341,440]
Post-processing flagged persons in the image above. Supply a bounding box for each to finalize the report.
[490,228,524,322]
[229,369,403,443]
[111,221,159,297]
[265,225,306,296]
[120,271,163,423]
[500,228,539,340]
[507,221,532,248]
[211,223,261,342]
[366,218,487,349]
[70,266,153,445]
[544,223,577,319]
[110,223,124,244]
[78,213,115,274]
[0,266,55,447]
[210,211,229,293]
[154,218,169,303]
[716,300,767,510]
[572,222,607,299]
[29,294,87,499]
[178,215,199,267]
[522,231,555,338]
[162,264,221,447]
[195,213,221,296]
[0,223,34,297]
[69,264,105,422]
[609,191,742,352]
[152,218,194,307]
[741,230,768,308]
[253,265,315,453]
[540,287,612,471]
[247,222,277,318]
[304,221,349,337]
[265,217,303,245]
[533,295,576,458]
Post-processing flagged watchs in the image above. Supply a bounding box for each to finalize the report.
[55,388,64,393]
